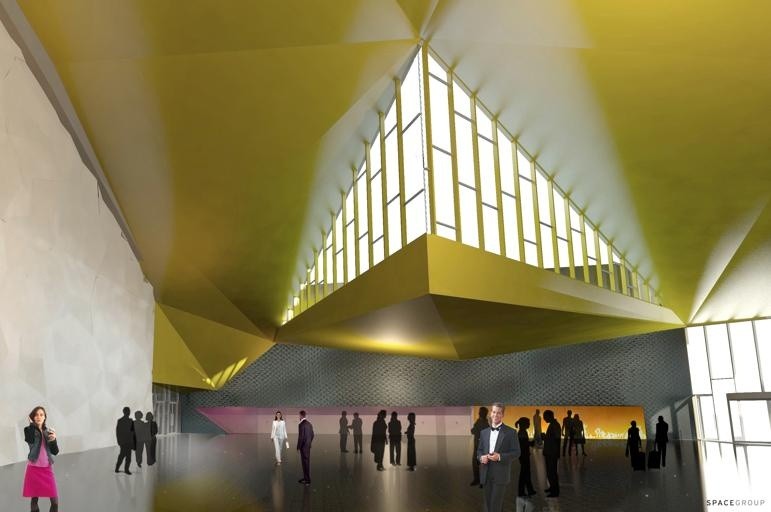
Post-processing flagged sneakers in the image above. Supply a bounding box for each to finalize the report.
[299,479,311,484]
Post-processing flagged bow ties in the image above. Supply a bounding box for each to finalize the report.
[490,425,501,432]
